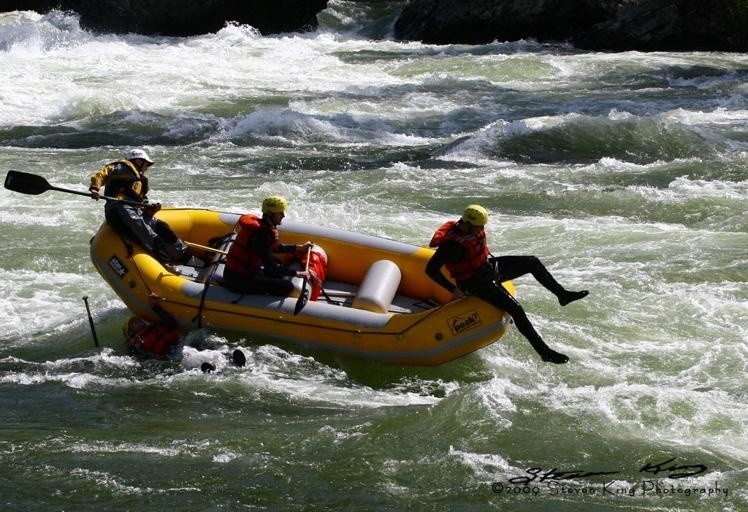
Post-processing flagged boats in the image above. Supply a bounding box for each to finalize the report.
[84,203,517,370]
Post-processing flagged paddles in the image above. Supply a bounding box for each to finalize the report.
[293,241,313,316]
[4,170,156,208]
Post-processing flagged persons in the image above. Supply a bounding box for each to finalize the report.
[89,148,192,266]
[120,292,247,374]
[423,203,589,365]
[224,195,315,297]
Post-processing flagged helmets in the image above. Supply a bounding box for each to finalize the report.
[262,196,286,214]
[126,149,154,167]
[461,205,488,225]
[123,316,135,339]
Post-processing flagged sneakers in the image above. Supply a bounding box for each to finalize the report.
[559,289,588,304]
[542,350,569,364]
